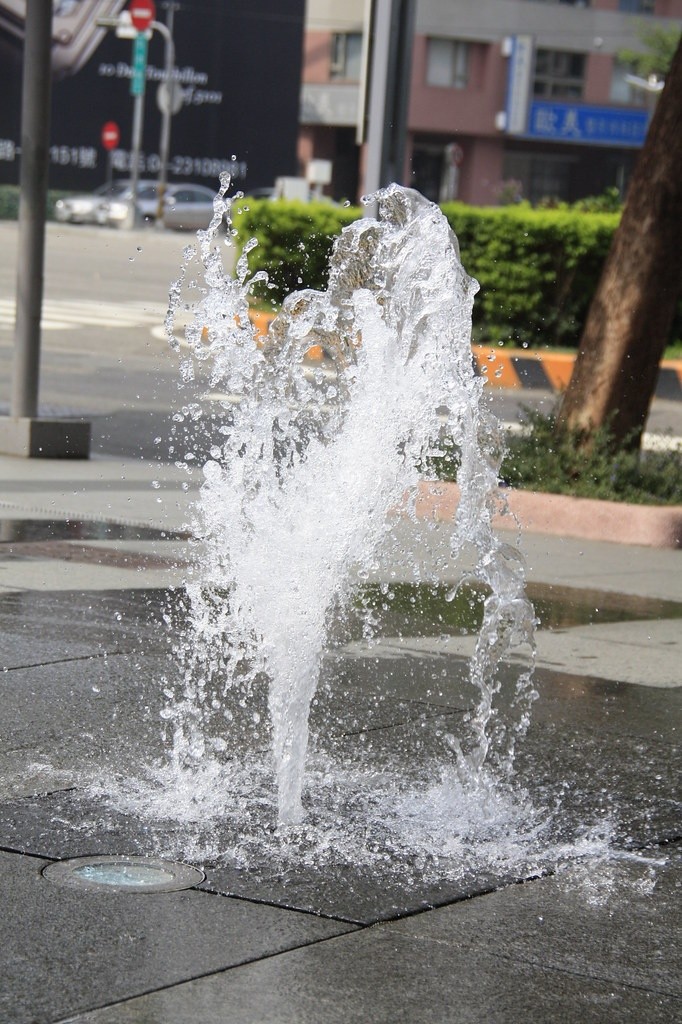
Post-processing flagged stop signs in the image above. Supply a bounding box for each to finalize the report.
[128,0,156,30]
[102,120,122,150]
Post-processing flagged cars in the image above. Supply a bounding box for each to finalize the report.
[54,178,226,231]
[243,187,337,207]
